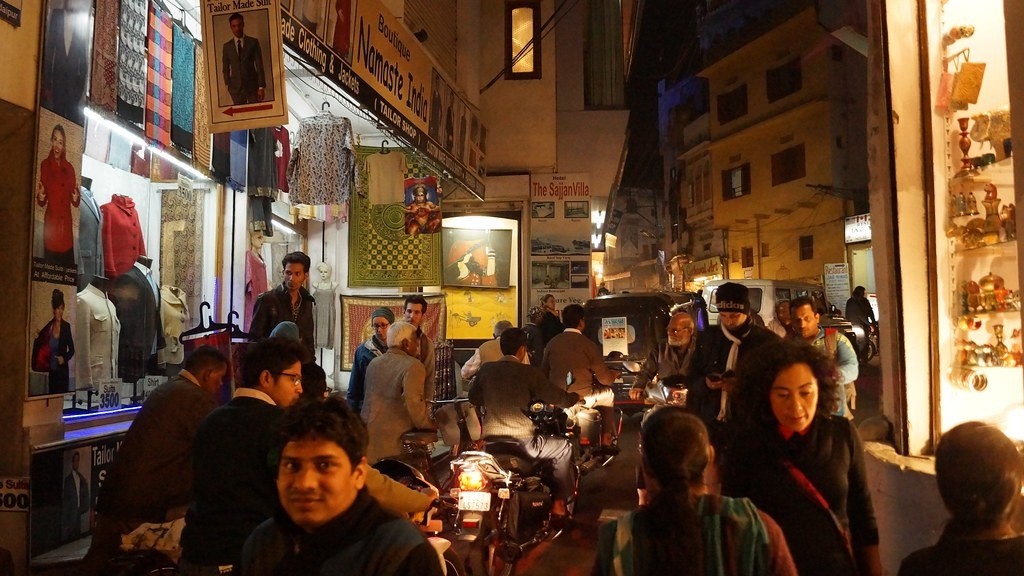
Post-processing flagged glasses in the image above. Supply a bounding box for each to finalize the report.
[280,372,302,386]
[371,322,391,329]
[666,327,690,335]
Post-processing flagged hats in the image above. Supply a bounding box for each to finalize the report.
[715,282,751,316]
[372,307,395,325]
[527,304,544,319]
[270,322,300,344]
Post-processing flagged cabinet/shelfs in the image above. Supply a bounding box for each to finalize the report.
[951,155,1022,371]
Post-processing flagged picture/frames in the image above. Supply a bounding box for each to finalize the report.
[441,227,512,289]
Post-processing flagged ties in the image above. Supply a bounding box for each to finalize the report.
[238,40,242,56]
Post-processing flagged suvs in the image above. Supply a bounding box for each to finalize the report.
[701,279,858,354]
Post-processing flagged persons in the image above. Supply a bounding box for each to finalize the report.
[245,226,269,333]
[39,288,75,393]
[41,0,86,123]
[311,263,339,348]
[85,253,1024,575]
[223,12,266,107]
[406,186,440,235]
[37,124,81,268]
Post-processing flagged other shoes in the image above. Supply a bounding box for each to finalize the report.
[550,509,575,531]
[601,444,621,456]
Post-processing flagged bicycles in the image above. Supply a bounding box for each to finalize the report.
[401,398,473,496]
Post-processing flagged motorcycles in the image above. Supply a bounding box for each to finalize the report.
[563,351,624,477]
[448,370,589,576]
[584,289,710,417]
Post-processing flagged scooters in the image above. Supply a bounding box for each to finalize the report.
[853,323,878,361]
[370,458,467,576]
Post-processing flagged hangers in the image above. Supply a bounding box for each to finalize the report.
[289,132,294,146]
[322,101,330,110]
[179,302,236,344]
[217,311,249,344]
[380,140,390,154]
